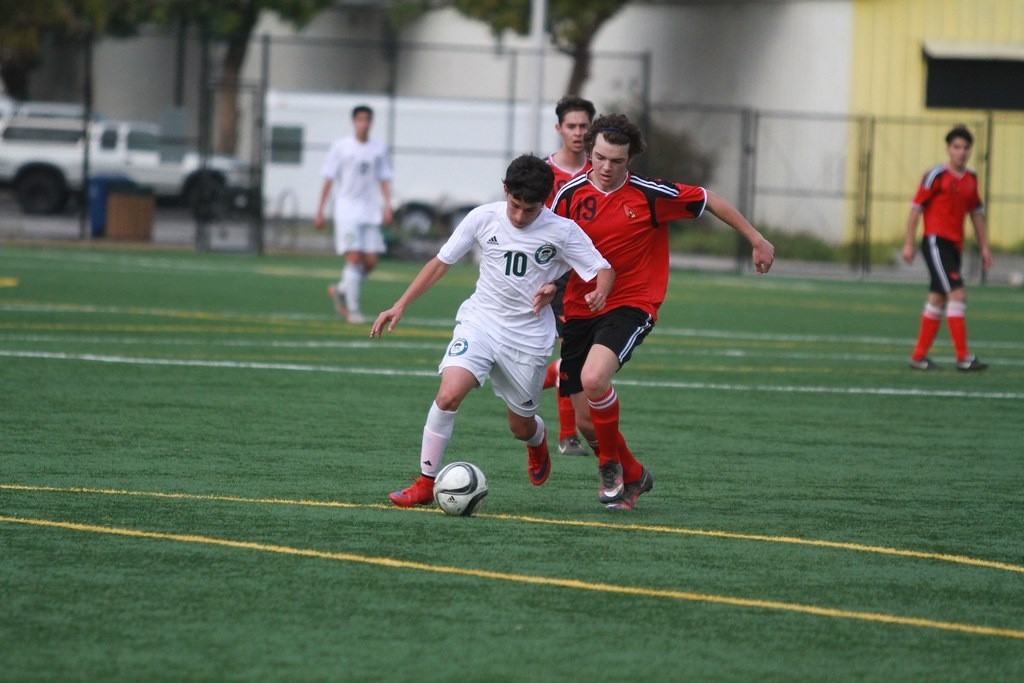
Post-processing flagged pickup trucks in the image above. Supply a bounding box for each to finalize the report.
[0,119,251,222]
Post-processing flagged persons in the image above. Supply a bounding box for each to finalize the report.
[902,125,993,372]
[370,154,616,509]
[542,93,596,457]
[316,105,394,326]
[533,113,774,508]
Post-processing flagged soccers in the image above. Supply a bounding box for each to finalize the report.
[433,462,489,517]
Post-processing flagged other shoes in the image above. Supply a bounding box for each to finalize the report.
[910,358,940,370]
[328,286,347,316]
[345,311,364,324]
[956,356,988,371]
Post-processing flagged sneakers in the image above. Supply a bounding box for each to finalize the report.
[389,476,438,508]
[559,435,590,456]
[598,462,625,503]
[606,467,653,511]
[527,428,551,485]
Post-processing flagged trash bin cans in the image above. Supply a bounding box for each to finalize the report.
[85,173,142,241]
[653,161,695,235]
[184,168,225,221]
[105,181,154,243]
